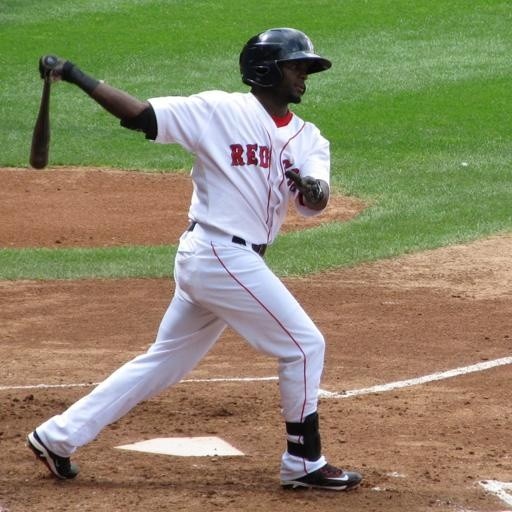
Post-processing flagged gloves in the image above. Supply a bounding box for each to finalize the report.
[38,54,99,95]
[285,170,324,206]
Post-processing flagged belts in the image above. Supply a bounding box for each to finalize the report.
[186,220,265,256]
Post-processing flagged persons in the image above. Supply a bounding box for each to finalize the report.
[24,26,364,493]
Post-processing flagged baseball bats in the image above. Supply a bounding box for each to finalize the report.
[30,54,57,170]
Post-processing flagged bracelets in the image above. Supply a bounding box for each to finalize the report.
[314,179,325,203]
[61,61,101,97]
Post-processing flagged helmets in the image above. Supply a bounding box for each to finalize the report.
[239,26,333,91]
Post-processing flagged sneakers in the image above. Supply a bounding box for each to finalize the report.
[281,463,365,491]
[26,427,78,480]
